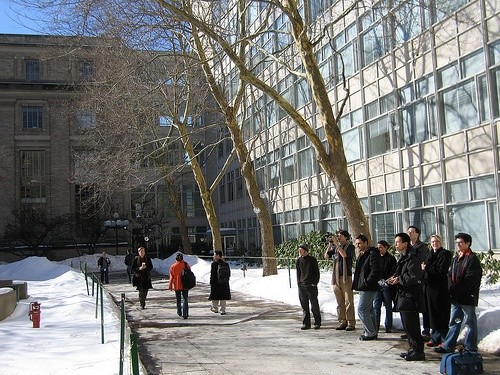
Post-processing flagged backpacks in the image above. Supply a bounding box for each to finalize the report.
[181,261,196,289]
[215,260,230,284]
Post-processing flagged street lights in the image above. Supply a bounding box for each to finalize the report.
[103,212,129,255]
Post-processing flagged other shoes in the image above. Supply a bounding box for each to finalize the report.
[335,323,346,330]
[345,326,354,331]
[421,334,431,341]
[404,352,425,361]
[314,325,320,329]
[301,325,310,330]
[400,350,411,358]
[426,341,440,346]
[433,346,449,353]
[361,334,377,341]
[401,335,409,340]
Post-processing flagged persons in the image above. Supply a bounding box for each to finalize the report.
[98,252,111,284]
[131,247,153,310]
[421,234,452,347]
[401,226,430,334]
[295,244,321,330]
[385,233,426,361]
[374,240,397,333]
[124,248,135,284]
[350,235,380,341]
[434,233,482,354]
[324,230,356,331]
[169,253,190,319]
[208,250,231,315]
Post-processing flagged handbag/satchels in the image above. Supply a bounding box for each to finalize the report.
[440,347,483,375]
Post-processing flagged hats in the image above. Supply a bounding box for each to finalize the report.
[299,243,309,252]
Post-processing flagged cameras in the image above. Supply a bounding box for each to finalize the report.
[321,232,333,243]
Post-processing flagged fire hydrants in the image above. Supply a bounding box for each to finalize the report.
[28,301,41,328]
